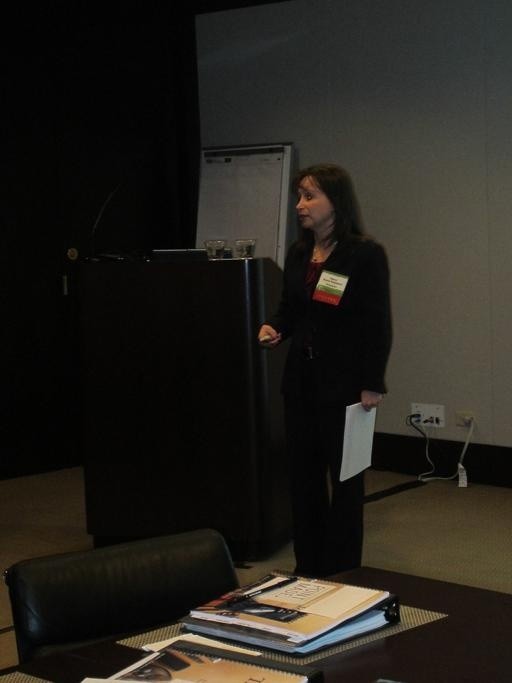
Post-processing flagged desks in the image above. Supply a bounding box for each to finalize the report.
[2,564,512,683]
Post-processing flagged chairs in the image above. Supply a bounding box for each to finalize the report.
[6,530,241,667]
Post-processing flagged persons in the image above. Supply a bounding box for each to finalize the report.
[255,161,396,578]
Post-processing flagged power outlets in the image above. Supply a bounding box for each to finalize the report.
[455,410,472,427]
[410,404,446,428]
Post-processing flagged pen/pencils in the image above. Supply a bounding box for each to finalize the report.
[259,332,284,342]
[236,577,297,601]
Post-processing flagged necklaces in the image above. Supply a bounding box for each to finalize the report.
[312,246,320,264]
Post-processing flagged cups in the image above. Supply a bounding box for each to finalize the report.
[204,239,226,259]
[235,240,258,259]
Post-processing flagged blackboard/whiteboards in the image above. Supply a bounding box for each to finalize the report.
[195,142,291,272]
